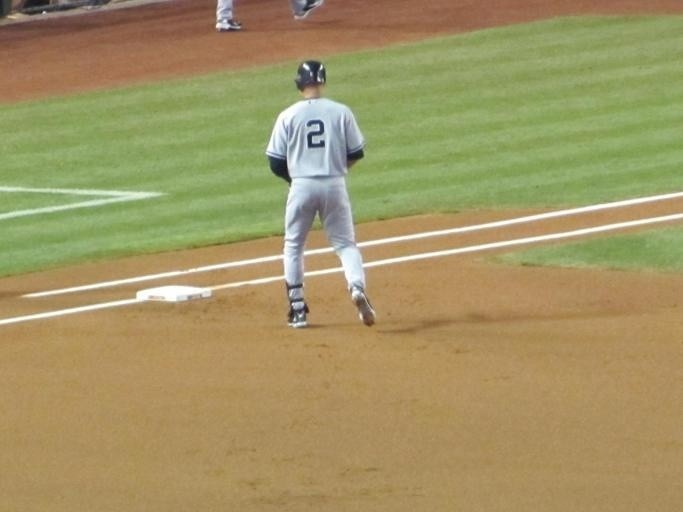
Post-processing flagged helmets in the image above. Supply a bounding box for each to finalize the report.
[295,61,326,91]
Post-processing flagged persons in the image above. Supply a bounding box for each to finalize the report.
[215,1,324,31]
[265,60,376,328]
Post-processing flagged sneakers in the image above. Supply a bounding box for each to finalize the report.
[351,286,377,326]
[294,0,323,19]
[216,19,241,30]
[287,309,308,328]
